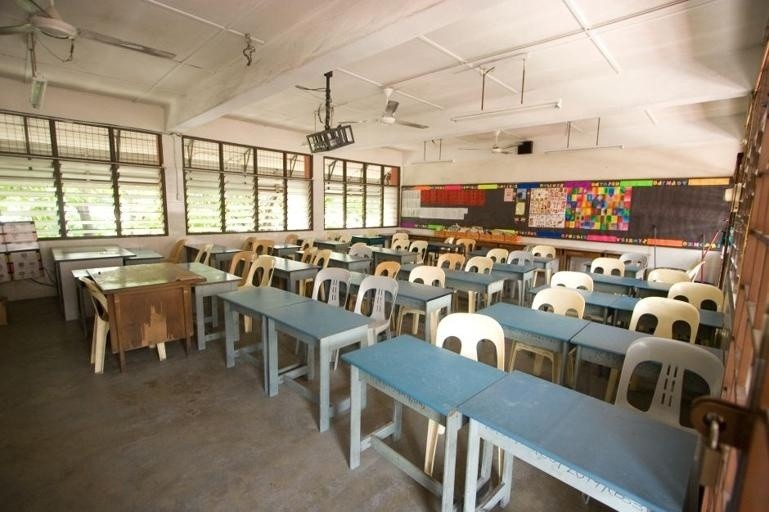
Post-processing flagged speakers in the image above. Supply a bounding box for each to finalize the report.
[518,141,532,154]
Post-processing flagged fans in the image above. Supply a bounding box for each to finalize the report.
[1,0,179,62]
[460,128,523,156]
[340,86,432,132]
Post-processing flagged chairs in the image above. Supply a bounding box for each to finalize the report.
[48,228,734,512]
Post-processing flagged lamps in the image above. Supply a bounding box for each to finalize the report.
[411,138,456,166]
[25,32,50,110]
[544,118,625,156]
[448,52,562,124]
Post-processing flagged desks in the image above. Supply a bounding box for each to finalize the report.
[50,244,137,322]
[216,286,313,394]
[457,369,699,512]
[570,321,723,393]
[341,334,509,512]
[124,249,164,266]
[86,262,207,374]
[265,300,377,435]
[474,302,591,384]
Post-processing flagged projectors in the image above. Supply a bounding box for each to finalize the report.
[314,137,340,150]
